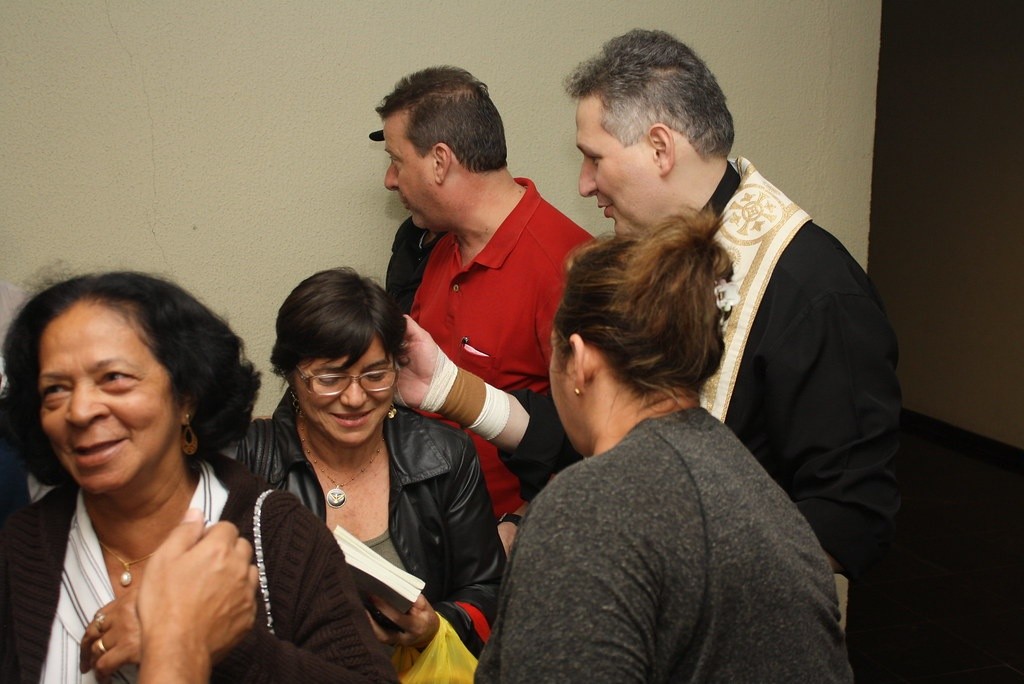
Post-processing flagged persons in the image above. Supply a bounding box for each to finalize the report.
[0,270,400,684]
[375,63,597,561]
[369,129,447,316]
[220,267,509,654]
[474,208,853,683]
[392,30,903,574]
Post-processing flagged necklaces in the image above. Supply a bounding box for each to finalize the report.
[98,540,154,585]
[302,420,385,508]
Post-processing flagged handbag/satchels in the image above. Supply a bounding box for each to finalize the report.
[389,611,478,684]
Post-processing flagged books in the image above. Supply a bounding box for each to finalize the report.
[334,526,426,616]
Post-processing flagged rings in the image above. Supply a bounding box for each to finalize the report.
[96,614,106,632]
[98,639,105,651]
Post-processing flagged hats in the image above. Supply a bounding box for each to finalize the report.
[369,130,385,141]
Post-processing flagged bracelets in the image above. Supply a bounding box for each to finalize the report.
[497,513,522,527]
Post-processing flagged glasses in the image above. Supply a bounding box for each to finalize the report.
[295,356,399,397]
